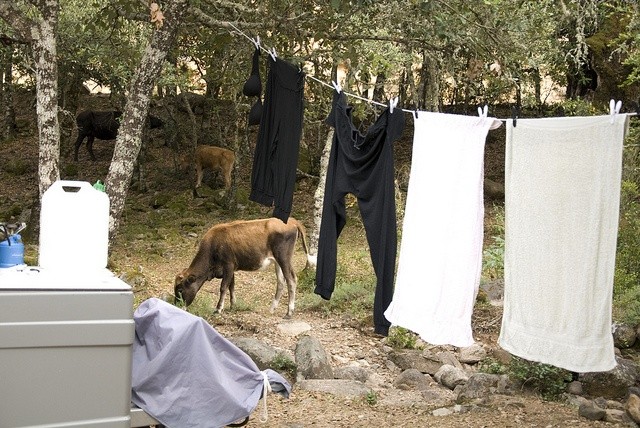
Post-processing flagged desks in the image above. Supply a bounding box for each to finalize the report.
[0,266,135,428]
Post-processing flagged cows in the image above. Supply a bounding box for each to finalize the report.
[174,216,312,319]
[73,109,167,165]
[174,144,236,196]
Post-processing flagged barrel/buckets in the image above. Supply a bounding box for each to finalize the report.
[38,180,110,274]
